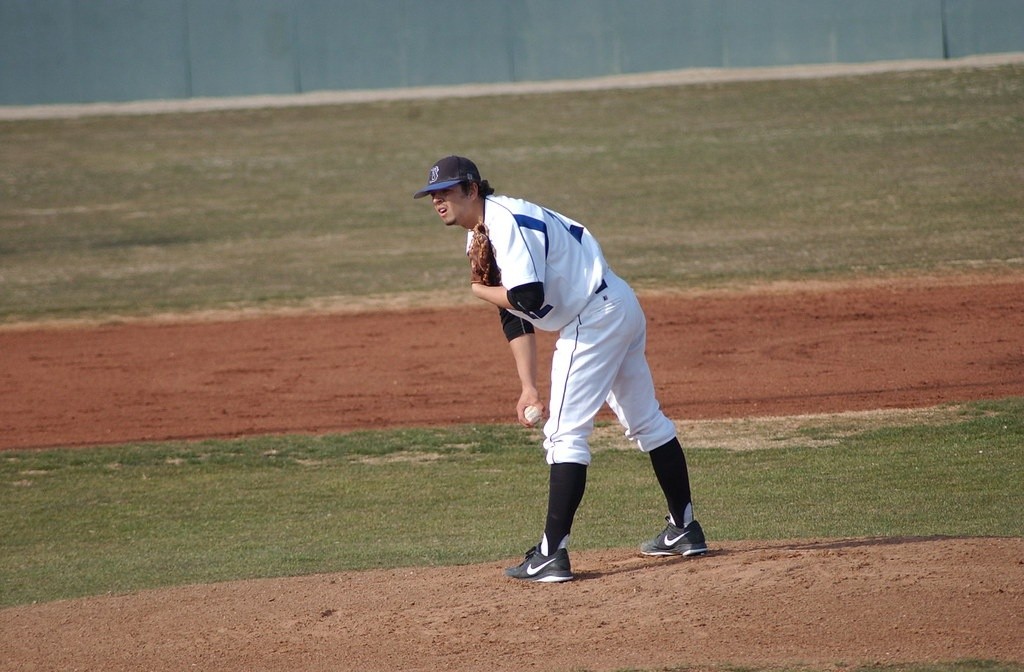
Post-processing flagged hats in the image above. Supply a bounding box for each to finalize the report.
[413,155,482,199]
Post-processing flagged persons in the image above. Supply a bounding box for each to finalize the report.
[413,154,712,586]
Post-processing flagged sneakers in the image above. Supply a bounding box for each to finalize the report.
[506,544,574,583]
[639,519,708,556]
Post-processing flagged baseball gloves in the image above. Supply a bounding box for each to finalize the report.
[467,221,503,289]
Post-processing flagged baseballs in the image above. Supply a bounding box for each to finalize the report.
[523,405,544,426]
